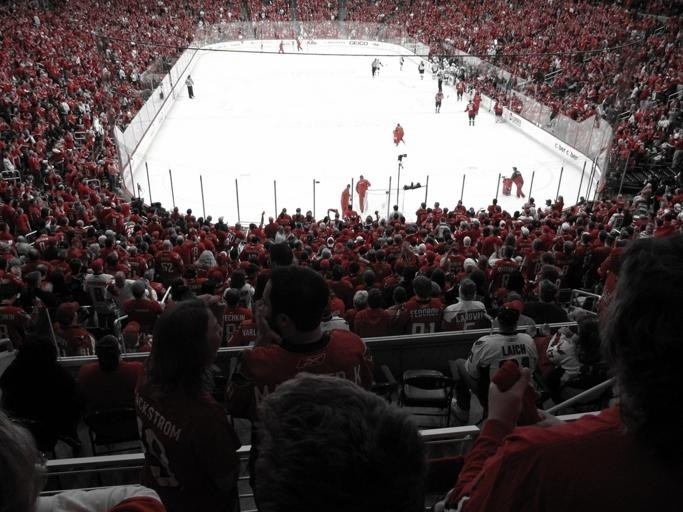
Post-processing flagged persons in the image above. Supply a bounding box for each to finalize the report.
[391,1,681,71]
[340,183,353,218]
[370,58,376,76]
[419,72,508,126]
[0,199,681,512]
[392,123,405,147]
[197,0,391,43]
[508,72,681,200]
[277,40,285,54]
[0,1,198,199]
[294,37,303,51]
[355,175,370,214]
[374,58,383,76]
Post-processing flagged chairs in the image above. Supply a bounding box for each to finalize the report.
[0,2,683,511]
